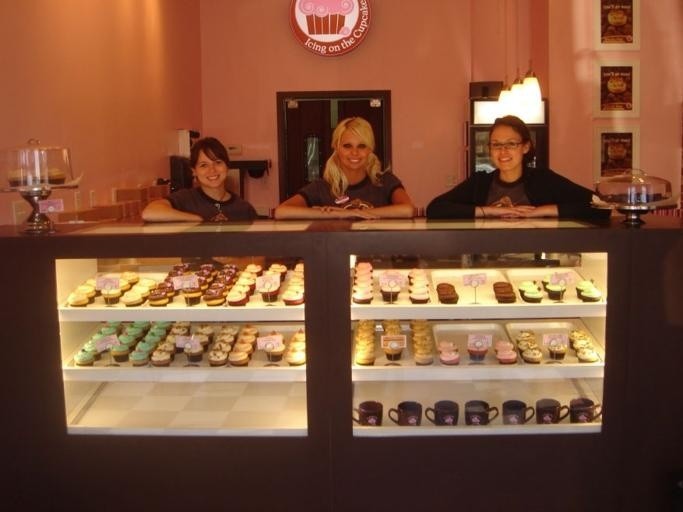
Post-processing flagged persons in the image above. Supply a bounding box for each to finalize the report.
[275,116,416,220]
[141,137,259,222]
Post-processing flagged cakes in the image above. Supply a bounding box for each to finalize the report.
[258,263,288,302]
[208,323,259,367]
[518,280,544,303]
[183,324,215,362]
[542,278,567,300]
[493,282,516,303]
[282,263,305,305]
[568,328,599,363]
[380,283,401,302]
[410,319,433,364]
[352,262,374,304]
[74,321,123,366]
[285,327,306,366]
[548,345,566,360]
[100,270,156,307]
[407,268,430,304]
[182,263,263,306]
[265,330,286,362]
[494,340,518,364]
[354,319,376,365]
[437,341,460,365]
[382,319,403,361]
[467,346,488,361]
[576,280,602,302]
[436,283,459,304]
[147,263,192,307]
[515,329,543,364]
[110,321,192,367]
[68,278,102,307]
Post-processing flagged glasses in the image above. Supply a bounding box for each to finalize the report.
[489,141,525,149]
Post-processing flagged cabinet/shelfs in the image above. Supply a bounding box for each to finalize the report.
[1,212,683,512]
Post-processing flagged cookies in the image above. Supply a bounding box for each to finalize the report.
[7,167,67,188]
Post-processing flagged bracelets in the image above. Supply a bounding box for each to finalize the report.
[426,115,613,222]
[479,206,487,217]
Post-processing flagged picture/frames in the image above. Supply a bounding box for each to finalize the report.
[594,126,639,183]
[591,60,640,118]
[594,0,640,52]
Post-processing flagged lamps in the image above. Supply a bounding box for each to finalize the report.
[508,0,524,118]
[498,0,511,118]
[520,0,543,119]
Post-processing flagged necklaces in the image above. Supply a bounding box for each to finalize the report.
[208,190,227,211]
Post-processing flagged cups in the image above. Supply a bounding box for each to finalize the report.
[502,400,535,425]
[353,400,384,426]
[465,400,498,425]
[536,398,570,424]
[425,400,460,426]
[569,398,602,423]
[388,400,422,426]
